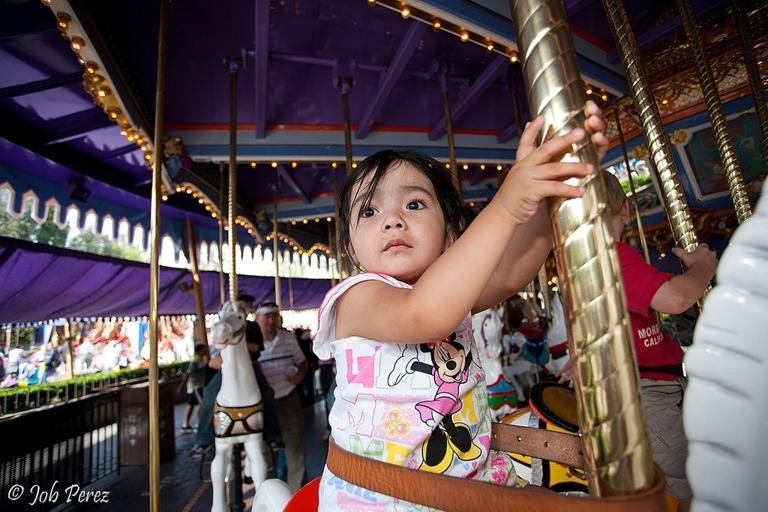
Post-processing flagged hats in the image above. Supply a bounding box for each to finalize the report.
[255,300,279,316]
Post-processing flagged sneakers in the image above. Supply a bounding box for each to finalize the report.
[181,424,199,433]
[188,444,212,458]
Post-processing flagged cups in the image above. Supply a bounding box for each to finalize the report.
[286,366,299,385]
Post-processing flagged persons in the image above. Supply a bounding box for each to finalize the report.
[597,168,719,501]
[175,343,212,434]
[293,325,335,443]
[308,97,624,511]
[250,299,310,494]
[0,319,150,389]
[186,294,282,462]
